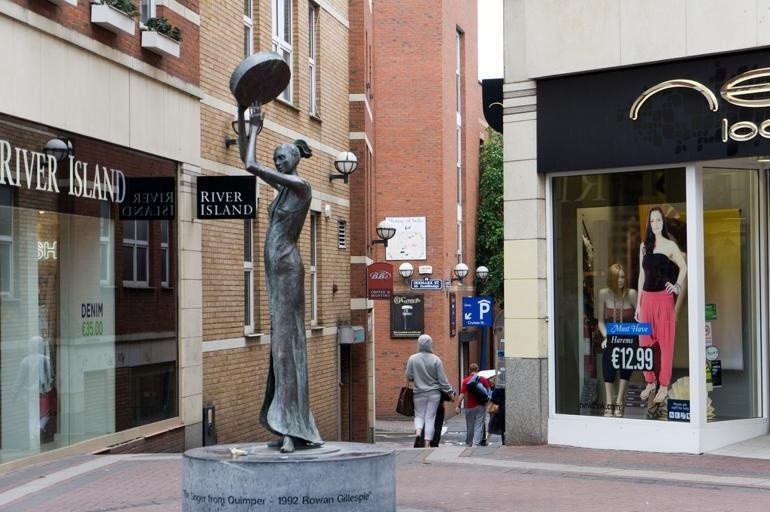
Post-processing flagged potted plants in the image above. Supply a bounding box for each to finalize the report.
[90,0,183,64]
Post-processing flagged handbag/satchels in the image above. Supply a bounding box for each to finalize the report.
[467,375,492,403]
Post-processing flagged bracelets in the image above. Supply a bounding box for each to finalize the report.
[674,282,680,290]
[603,336,608,339]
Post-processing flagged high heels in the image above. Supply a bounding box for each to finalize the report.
[396,387,414,416]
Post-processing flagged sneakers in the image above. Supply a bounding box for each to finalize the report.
[415,436,421,448]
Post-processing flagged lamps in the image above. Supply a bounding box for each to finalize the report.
[329,151,359,184]
[39,138,74,171]
[369,218,490,285]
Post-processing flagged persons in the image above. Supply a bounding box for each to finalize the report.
[597,264,639,418]
[456,364,490,446]
[235,97,324,453]
[420,389,456,447]
[634,207,687,402]
[405,333,455,448]
[482,382,505,446]
